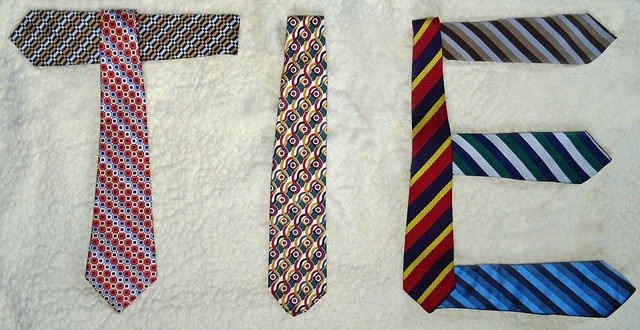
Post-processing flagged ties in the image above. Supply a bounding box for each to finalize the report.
[85,9,159,317]
[451,130,613,184]
[434,259,635,317]
[269,16,326,318]
[441,13,616,65]
[404,17,455,314]
[7,9,240,68]
